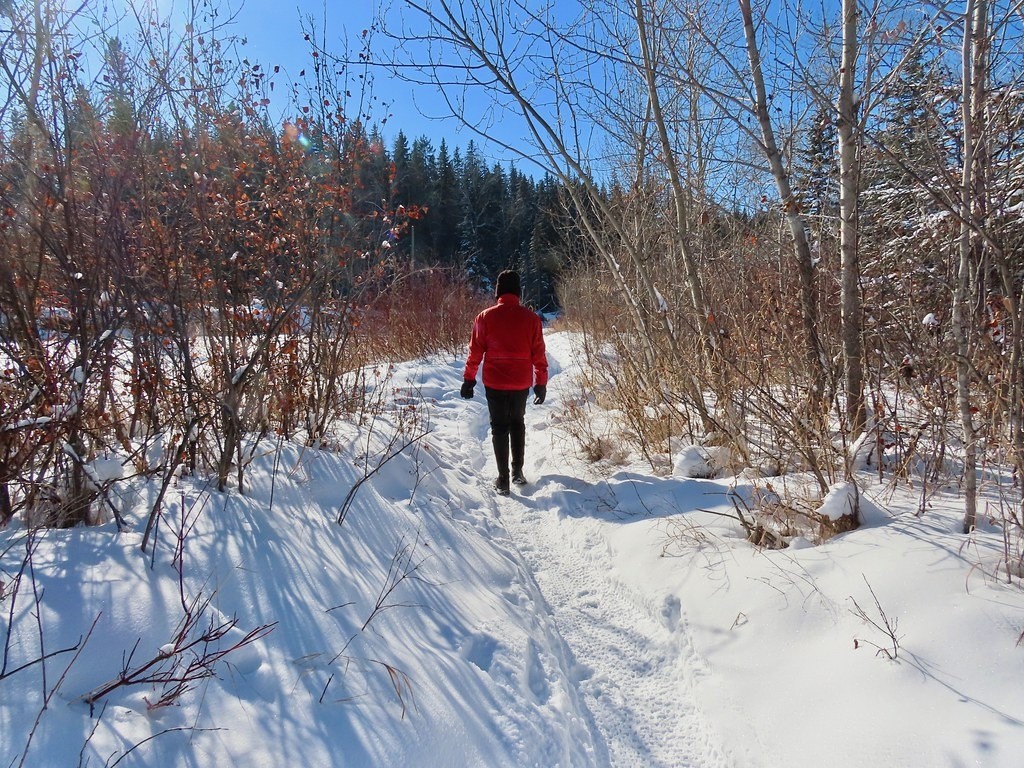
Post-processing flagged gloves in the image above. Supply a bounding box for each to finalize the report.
[533,385,546,405]
[461,379,476,399]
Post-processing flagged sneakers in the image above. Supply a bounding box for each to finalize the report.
[491,476,510,495]
[512,470,526,484]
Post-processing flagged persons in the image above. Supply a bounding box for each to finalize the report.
[460,270,548,495]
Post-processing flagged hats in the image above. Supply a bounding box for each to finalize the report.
[494,269,521,300]
